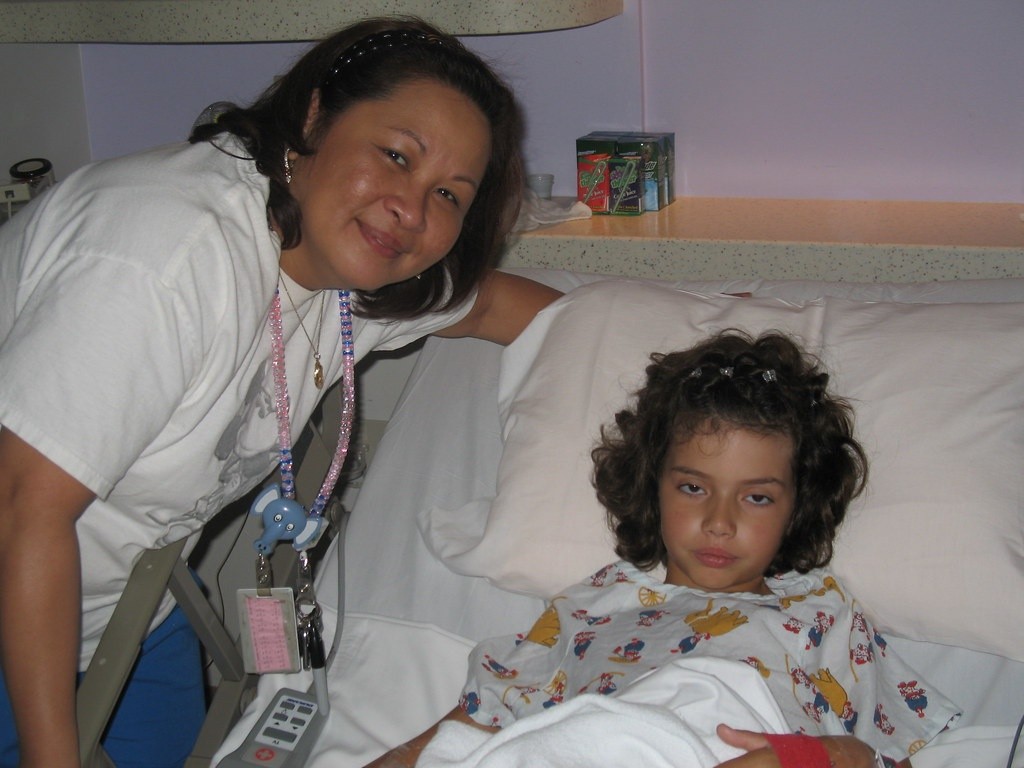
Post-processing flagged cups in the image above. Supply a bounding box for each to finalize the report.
[528,173,554,203]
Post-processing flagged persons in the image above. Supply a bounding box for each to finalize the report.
[361,324,964,768]
[1,11,752,768]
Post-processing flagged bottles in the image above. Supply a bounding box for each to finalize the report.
[8,157,55,198]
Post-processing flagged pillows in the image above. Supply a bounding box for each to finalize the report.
[416,281,1024,667]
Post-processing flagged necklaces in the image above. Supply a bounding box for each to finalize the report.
[267,213,326,389]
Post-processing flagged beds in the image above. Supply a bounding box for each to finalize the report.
[216,265,1024,768]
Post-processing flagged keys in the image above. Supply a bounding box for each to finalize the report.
[296,617,330,716]
